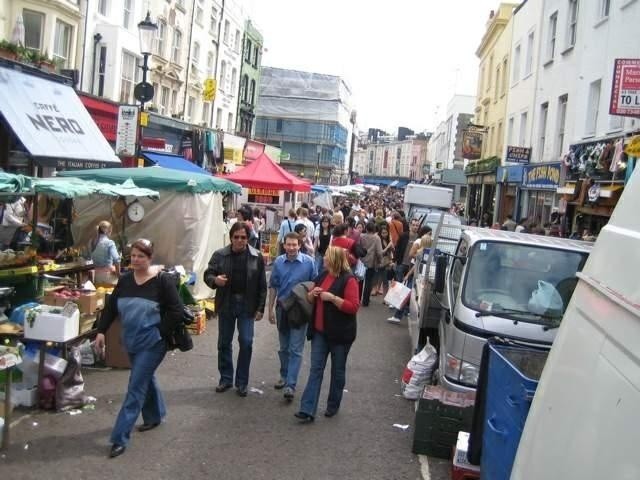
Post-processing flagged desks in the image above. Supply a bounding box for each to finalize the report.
[0,327,98,450]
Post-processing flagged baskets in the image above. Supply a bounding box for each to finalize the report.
[412,398,475,459]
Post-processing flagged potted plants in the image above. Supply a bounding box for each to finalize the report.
[0,38,65,72]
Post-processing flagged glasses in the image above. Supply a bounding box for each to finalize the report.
[233,234,247,239]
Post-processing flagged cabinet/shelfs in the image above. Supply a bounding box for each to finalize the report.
[0,191,95,297]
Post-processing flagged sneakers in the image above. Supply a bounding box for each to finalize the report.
[274,379,286,389]
[387,317,401,323]
[284,386,294,398]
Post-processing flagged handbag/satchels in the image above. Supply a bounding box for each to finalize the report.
[354,258,367,280]
[384,280,411,312]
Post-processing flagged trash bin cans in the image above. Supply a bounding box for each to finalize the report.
[480,344,551,480]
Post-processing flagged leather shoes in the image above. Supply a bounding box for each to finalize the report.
[111,444,125,457]
[216,382,233,392]
[139,423,158,431]
[239,385,248,396]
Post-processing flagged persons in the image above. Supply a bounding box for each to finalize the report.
[93,237,195,458]
[462,210,595,242]
[203,221,268,397]
[268,232,319,400]
[223,182,435,327]
[1,193,75,247]
[87,219,122,288]
[294,245,360,423]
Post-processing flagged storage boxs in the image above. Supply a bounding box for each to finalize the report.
[24,286,132,369]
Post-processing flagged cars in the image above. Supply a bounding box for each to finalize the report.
[415,213,463,245]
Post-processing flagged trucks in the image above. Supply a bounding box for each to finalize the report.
[402,182,455,221]
[230,183,311,218]
[405,223,594,397]
[507,158,640,480]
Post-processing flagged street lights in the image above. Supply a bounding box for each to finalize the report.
[315,139,323,183]
[133,10,158,172]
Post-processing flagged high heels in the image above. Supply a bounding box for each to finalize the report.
[295,412,314,421]
